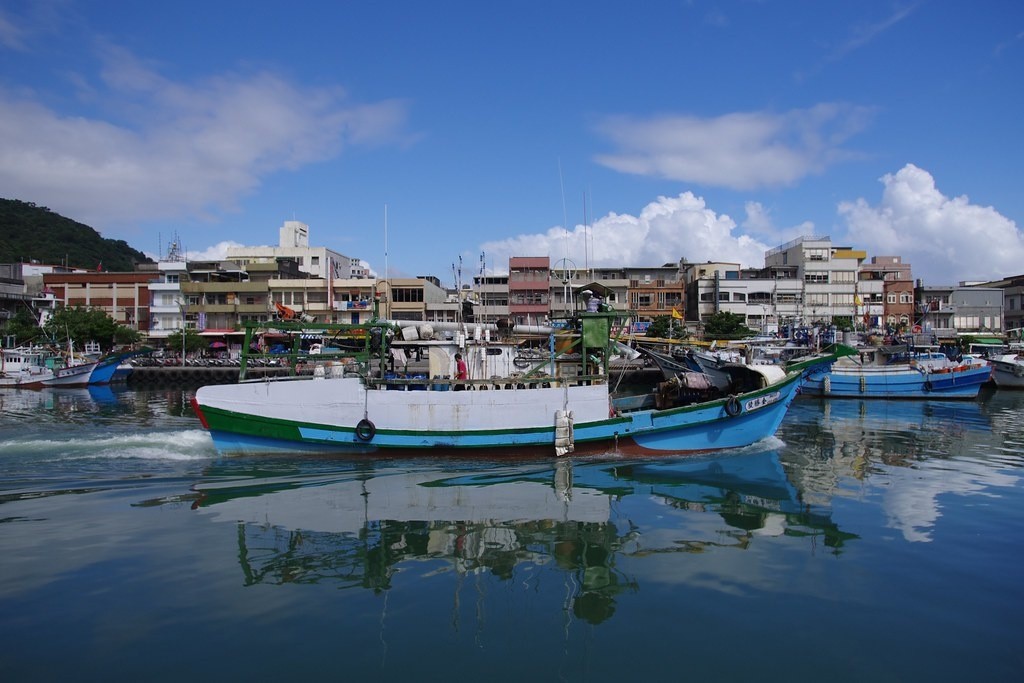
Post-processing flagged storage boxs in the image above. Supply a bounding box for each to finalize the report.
[385,374,451,392]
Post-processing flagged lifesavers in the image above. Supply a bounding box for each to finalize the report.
[725,398,743,417]
[356,419,376,441]
[128,369,236,380]
[127,380,193,392]
[925,381,932,391]
[794,330,804,339]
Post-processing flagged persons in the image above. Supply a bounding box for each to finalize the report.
[452,354,467,390]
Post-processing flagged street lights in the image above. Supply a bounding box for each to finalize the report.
[933,297,941,345]
[173,300,195,367]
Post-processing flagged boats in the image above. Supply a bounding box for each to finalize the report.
[189,202,860,459]
[694,309,1024,401]
[0,296,164,388]
